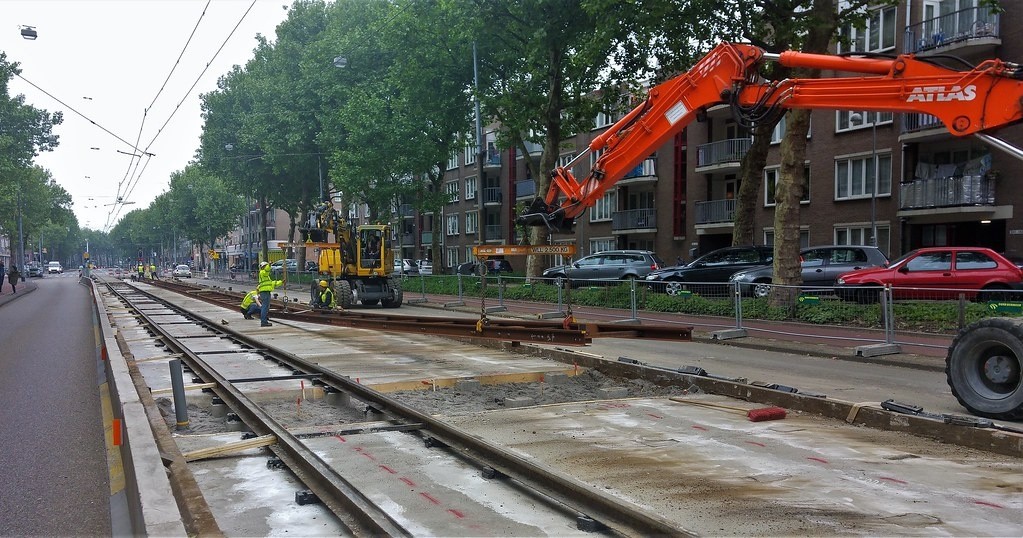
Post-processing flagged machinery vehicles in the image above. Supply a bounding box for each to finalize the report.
[514,42,1022,418]
[298,202,403,310]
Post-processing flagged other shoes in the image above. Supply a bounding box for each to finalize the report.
[244,314,254,319]
[261,323,272,327]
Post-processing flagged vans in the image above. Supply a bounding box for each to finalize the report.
[48,261,60,274]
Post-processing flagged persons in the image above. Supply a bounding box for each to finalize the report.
[7,264,21,295]
[137,264,145,281]
[240,286,263,321]
[257,259,287,328]
[363,234,381,258]
[0,260,6,296]
[149,262,159,282]
[308,280,338,313]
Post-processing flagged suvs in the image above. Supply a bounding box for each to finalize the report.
[394,259,419,274]
[645,244,772,295]
[728,246,889,299]
[543,249,666,288]
[416,260,433,275]
[485,259,513,283]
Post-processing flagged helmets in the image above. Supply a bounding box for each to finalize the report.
[319,281,328,287]
[260,262,268,268]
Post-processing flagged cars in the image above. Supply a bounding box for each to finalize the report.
[21,263,50,273]
[173,265,192,278]
[833,246,1023,306]
[60,265,64,273]
[270,259,318,272]
